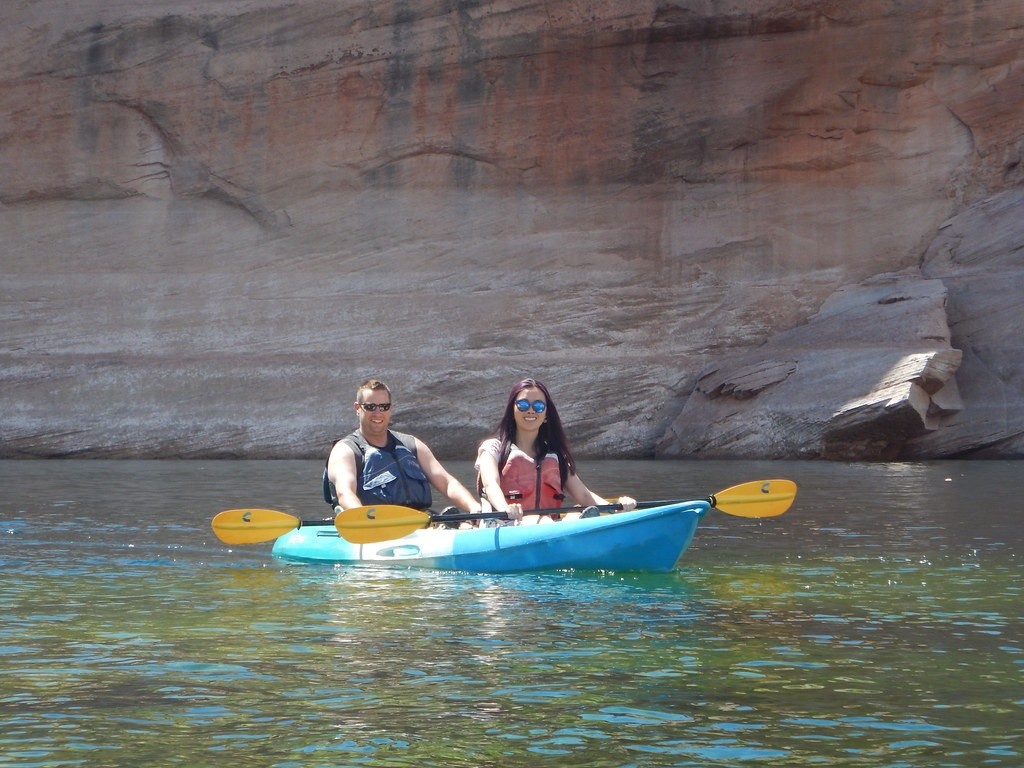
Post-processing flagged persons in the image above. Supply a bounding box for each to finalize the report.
[329,380,482,530]
[474,378,637,528]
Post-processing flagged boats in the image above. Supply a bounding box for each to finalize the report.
[268,500,712,578]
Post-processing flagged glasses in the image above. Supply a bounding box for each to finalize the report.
[515,399,546,413]
[357,403,391,412]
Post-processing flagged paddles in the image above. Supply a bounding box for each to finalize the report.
[332,478,799,546]
[209,495,630,547]
